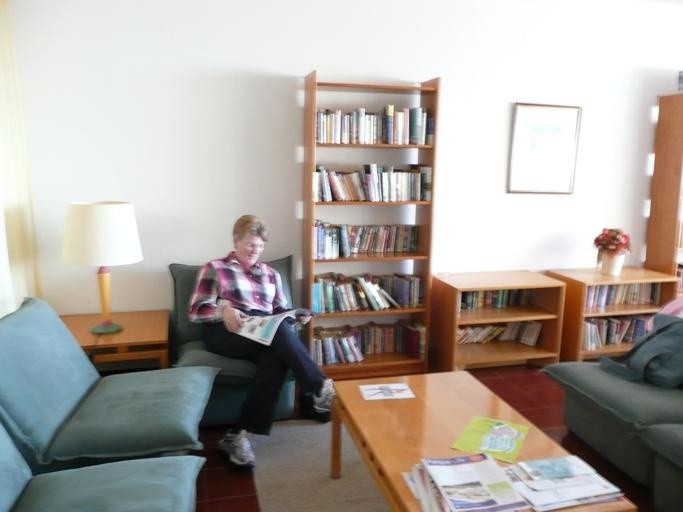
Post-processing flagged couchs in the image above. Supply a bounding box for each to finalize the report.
[538,314,682,511]
[169,255,296,428]
[0,298,222,512]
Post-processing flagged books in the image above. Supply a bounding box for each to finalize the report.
[457,290,544,348]
[582,283,661,350]
[233,307,310,346]
[310,104,435,367]
[399,453,624,512]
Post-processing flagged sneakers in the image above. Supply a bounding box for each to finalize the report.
[218,428,255,467]
[313,377,336,412]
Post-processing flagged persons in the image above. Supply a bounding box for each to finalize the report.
[188,215,335,467]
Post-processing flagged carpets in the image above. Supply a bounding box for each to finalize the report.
[251,418,396,510]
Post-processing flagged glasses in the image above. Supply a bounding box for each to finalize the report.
[245,242,265,250]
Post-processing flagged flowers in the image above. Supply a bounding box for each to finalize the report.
[593,228,634,264]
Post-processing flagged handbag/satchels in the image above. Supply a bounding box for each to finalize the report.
[598,313,683,390]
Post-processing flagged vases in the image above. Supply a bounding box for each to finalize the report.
[602,253,626,275]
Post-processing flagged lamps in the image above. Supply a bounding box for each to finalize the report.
[61,201,144,336]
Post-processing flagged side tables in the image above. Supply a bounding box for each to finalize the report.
[59,310,170,369]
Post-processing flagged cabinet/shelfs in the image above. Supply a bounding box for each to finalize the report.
[302,71,441,382]
[546,266,680,362]
[433,272,567,371]
[645,93,683,303]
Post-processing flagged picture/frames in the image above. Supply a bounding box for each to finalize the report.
[508,102,582,195]
